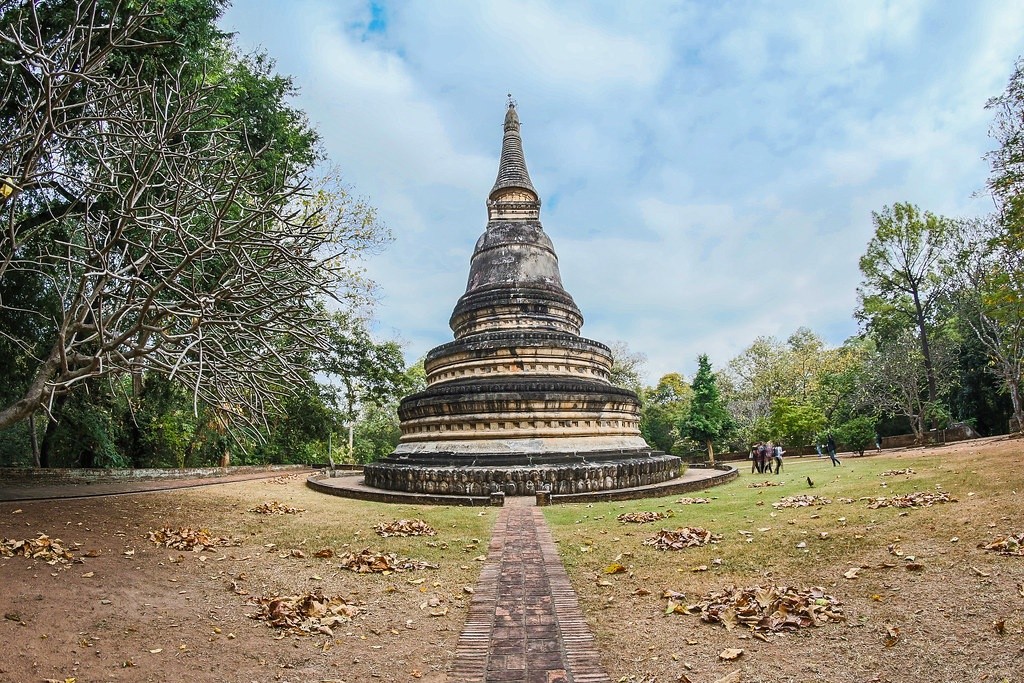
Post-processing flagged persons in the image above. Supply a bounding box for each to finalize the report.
[751,441,786,475]
[873,430,881,453]
[825,435,840,467]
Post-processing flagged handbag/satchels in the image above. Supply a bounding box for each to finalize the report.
[749,451,753,458]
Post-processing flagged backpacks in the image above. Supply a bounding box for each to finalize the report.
[771,449,776,457]
